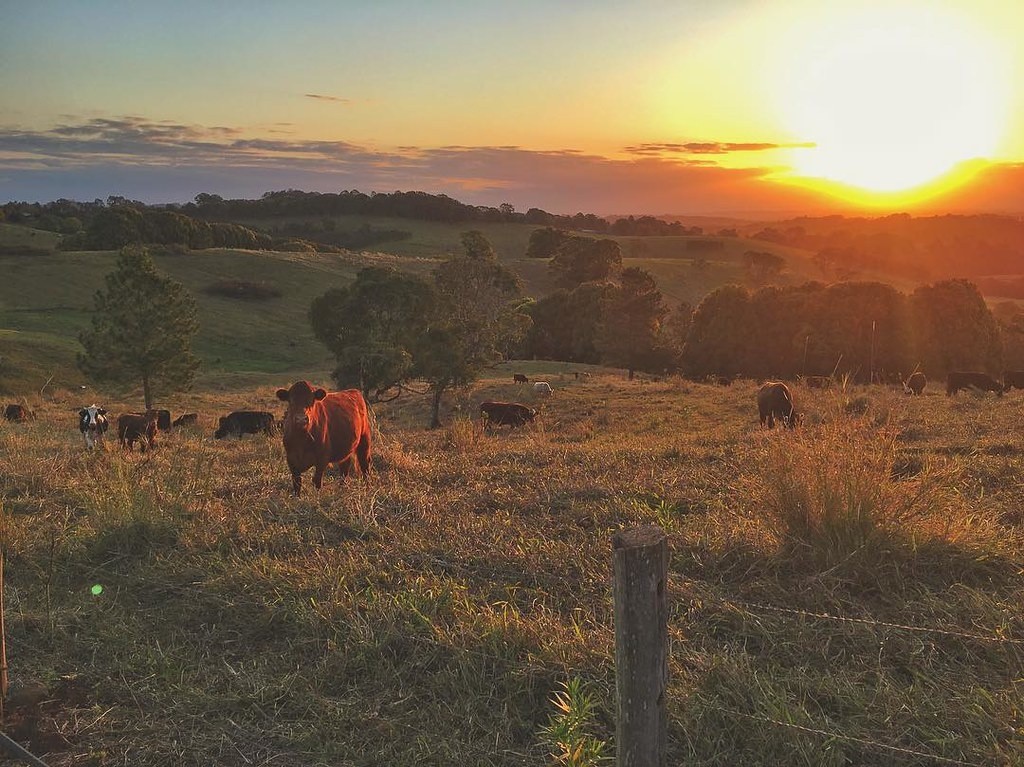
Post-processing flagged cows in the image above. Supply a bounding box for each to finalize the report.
[3,405,28,426]
[117,409,198,455]
[214,410,275,442]
[756,370,1012,429]
[275,380,373,499]
[79,403,109,453]
[513,374,554,400]
[480,401,537,432]
